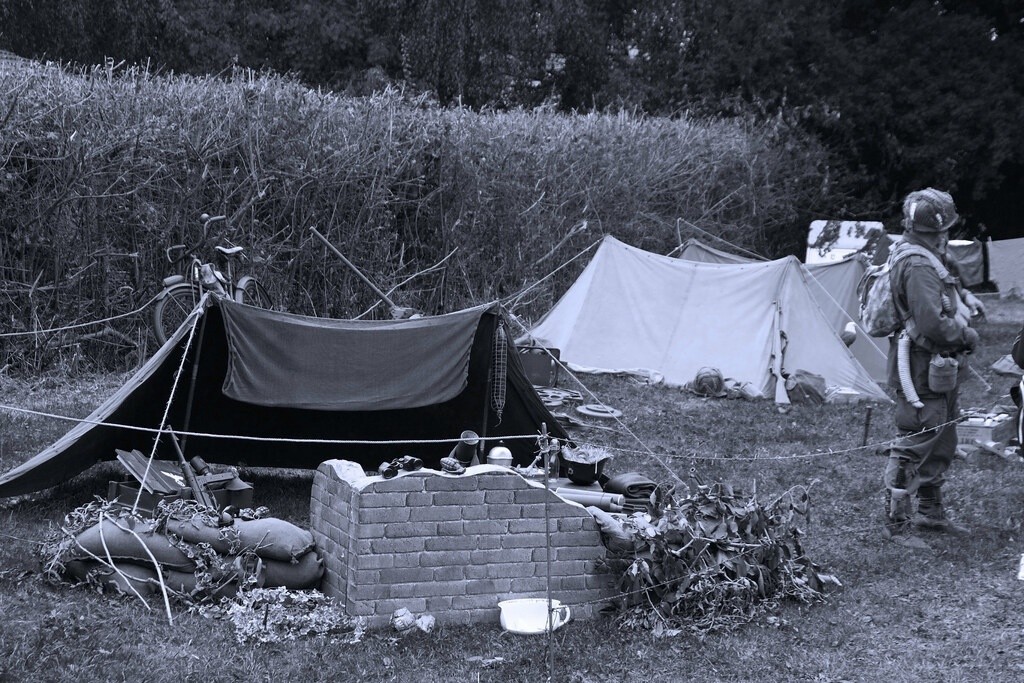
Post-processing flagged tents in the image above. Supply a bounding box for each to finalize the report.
[0,285,613,526]
[515,232,897,407]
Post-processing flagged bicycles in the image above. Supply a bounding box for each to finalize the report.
[152,214,276,350]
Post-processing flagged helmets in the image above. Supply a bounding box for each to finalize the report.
[559,445,610,485]
[900,187,959,232]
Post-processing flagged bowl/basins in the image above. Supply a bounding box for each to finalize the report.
[560,453,608,485]
[498,598,571,636]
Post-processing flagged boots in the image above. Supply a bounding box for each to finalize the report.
[882,506,931,552]
[915,501,970,536]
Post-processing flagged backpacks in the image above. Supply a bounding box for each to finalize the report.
[856,245,959,337]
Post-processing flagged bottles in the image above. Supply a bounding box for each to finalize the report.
[548,438,561,489]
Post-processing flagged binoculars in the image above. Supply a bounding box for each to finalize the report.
[378,454,424,479]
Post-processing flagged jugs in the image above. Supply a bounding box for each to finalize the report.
[929,351,957,393]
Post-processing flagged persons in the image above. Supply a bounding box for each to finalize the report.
[1009,327,1024,458]
[883,185,987,536]
